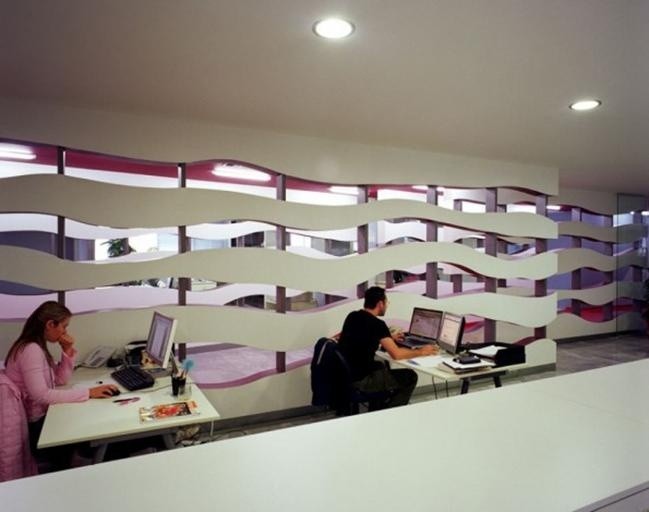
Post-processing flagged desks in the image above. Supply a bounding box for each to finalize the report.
[375,344,531,394]
[36,359,221,467]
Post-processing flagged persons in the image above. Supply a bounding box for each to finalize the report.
[336,287,439,412]
[4,300,121,470]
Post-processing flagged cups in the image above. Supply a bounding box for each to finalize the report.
[178,385,192,401]
[172,376,186,396]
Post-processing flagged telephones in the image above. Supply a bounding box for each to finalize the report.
[81,345,117,368]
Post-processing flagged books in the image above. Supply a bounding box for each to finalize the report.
[407,345,508,375]
[136,398,203,426]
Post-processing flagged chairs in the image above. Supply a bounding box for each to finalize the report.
[313,338,391,416]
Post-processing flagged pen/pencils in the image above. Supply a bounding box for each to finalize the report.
[170,353,193,380]
[113,397,139,405]
